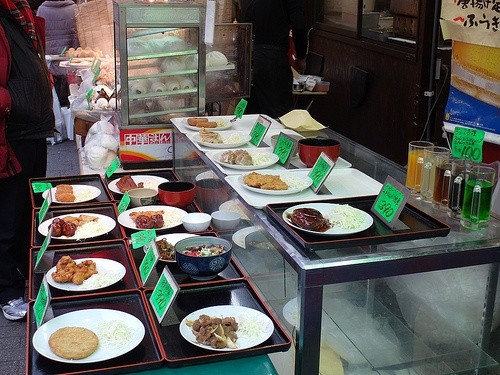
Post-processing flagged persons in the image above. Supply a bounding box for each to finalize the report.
[35,0,79,107]
[235,0,307,120]
[0,0,55,321]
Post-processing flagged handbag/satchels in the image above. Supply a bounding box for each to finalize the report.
[52,87,67,142]
[61,106,75,140]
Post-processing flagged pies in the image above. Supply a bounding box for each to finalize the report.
[48,327,99,359]
[244,172,288,191]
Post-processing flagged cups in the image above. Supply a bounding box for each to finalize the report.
[405,140,496,233]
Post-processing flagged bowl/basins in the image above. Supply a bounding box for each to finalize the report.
[174,235,233,280]
[128,189,159,206]
[270,134,303,156]
[292,83,306,92]
[245,231,283,270]
[159,180,195,209]
[183,212,212,234]
[299,138,341,168]
[194,177,241,233]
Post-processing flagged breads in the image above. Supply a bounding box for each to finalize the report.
[97,60,115,88]
[55,184,76,202]
[64,46,100,58]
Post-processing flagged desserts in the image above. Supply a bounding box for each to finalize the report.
[135,214,164,229]
[291,208,326,230]
[115,175,137,193]
[48,217,78,236]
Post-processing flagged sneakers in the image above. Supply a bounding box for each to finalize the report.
[0,296,29,320]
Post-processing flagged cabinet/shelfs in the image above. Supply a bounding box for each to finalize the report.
[308,0,442,168]
[111,0,206,126]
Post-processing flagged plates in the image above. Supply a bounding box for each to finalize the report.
[116,204,189,231]
[238,170,313,196]
[31,184,146,363]
[179,305,275,351]
[142,232,200,263]
[108,175,169,196]
[232,225,264,249]
[195,170,219,182]
[213,149,280,170]
[181,119,232,131]
[192,131,252,149]
[282,202,373,236]
[218,198,250,220]
